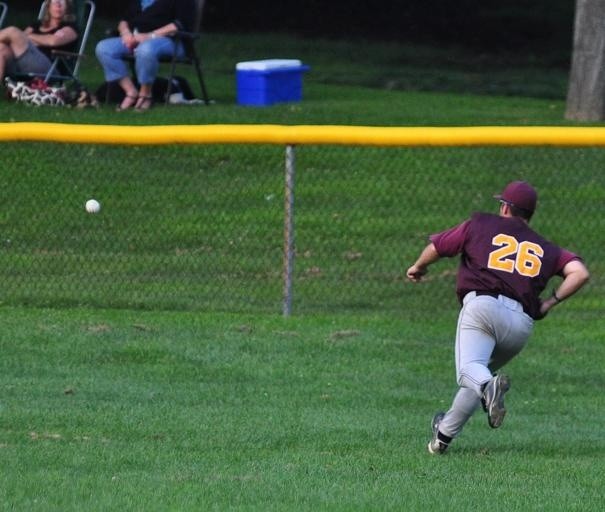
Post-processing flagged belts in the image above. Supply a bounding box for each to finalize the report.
[475,290,532,316]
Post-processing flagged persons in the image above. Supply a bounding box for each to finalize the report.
[407,181,590,454]
[0,0,81,83]
[94,0,197,114]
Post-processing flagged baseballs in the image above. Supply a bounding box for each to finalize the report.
[86,199,99,212]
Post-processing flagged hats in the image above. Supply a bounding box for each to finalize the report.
[493,181,538,213]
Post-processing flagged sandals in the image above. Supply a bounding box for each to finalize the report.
[117,95,153,112]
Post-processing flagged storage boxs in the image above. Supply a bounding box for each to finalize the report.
[236,60,309,106]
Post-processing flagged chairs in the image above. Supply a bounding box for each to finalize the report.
[0,1,8,26]
[106,0,211,107]
[9,0,96,104]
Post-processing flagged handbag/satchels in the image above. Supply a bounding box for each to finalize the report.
[4,74,68,108]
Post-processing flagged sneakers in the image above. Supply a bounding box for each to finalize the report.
[481,374,511,430]
[428,412,449,455]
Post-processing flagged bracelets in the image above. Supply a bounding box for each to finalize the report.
[552,288,560,302]
[151,31,156,39]
[120,29,130,36]
